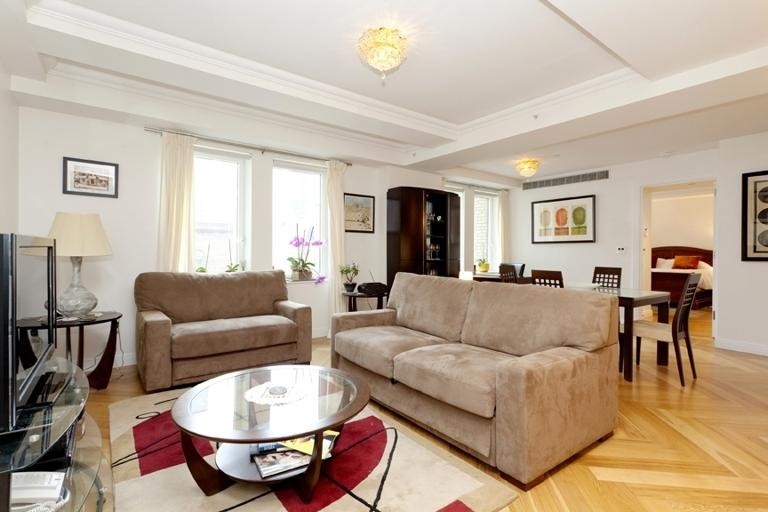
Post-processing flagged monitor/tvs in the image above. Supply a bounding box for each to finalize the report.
[0,230,72,430]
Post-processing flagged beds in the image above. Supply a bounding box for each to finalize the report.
[651,246,713,310]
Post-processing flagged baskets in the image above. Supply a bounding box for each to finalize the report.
[291,268,312,281]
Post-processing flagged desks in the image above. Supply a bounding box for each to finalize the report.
[0,357,114,512]
[473,273,531,283]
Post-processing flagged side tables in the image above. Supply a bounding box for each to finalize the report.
[17,312,122,388]
[342,292,387,312]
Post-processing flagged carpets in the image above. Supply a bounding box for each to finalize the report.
[109,383,521,512]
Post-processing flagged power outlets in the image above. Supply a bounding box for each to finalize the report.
[31,337,41,352]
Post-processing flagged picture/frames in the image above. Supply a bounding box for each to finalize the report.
[531,194,596,243]
[343,192,375,233]
[741,170,768,261]
[63,156,118,198]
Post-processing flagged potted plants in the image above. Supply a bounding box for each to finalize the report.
[339,263,360,292]
[477,257,490,271]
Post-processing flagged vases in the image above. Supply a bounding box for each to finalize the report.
[293,271,311,282]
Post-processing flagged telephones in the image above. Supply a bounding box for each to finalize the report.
[10,472,65,505]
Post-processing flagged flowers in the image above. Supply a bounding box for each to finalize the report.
[290,225,326,285]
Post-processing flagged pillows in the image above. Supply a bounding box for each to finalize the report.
[672,256,701,269]
[656,258,675,268]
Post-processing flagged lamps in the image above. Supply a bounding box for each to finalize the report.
[40,211,112,319]
[356,27,407,80]
[515,159,539,178]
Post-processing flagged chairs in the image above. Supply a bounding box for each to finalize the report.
[497,263,701,385]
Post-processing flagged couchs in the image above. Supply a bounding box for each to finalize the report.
[134,271,311,393]
[330,272,619,486]
